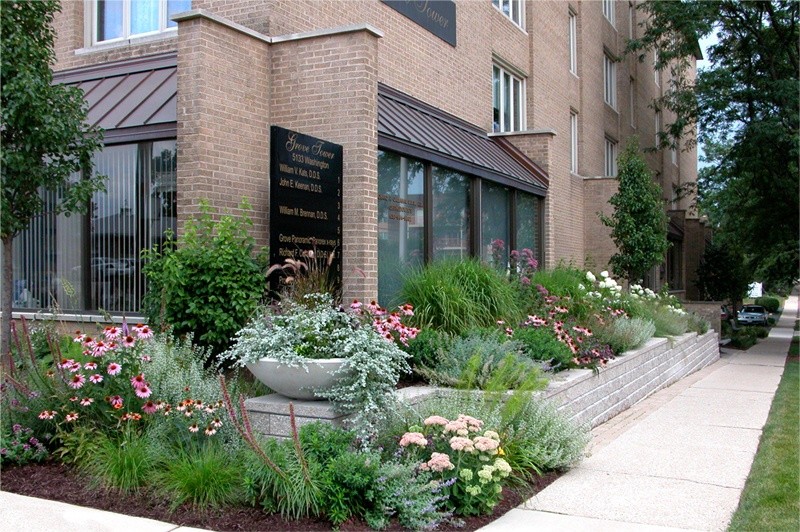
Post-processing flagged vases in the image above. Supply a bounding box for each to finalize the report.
[244,357,349,399]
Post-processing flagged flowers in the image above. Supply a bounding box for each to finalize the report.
[217,293,419,406]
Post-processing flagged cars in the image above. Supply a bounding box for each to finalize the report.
[72,257,139,280]
[736,305,773,327]
